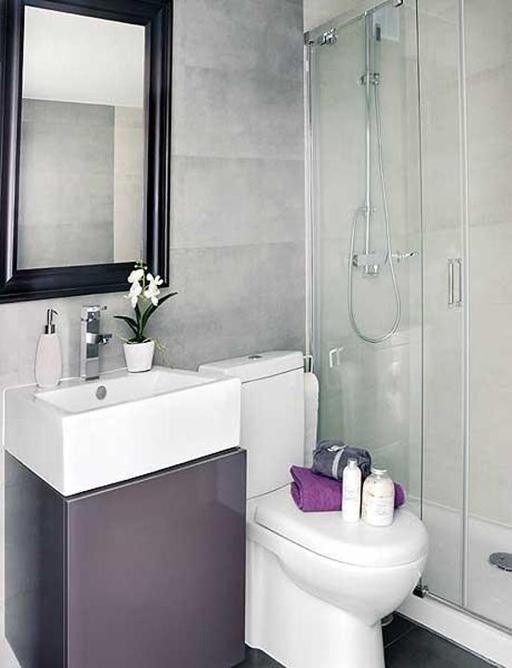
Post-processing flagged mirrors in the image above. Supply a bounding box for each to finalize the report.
[2,3,178,307]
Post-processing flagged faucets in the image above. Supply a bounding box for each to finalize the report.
[79,304,113,381]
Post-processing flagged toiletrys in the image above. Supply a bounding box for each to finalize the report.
[363,466,395,529]
[340,458,361,521]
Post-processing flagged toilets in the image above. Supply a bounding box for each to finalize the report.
[198,348,430,667]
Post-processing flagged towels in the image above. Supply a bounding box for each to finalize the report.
[291,466,408,512]
[313,442,372,480]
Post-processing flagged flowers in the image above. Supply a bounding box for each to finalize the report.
[112,258,178,341]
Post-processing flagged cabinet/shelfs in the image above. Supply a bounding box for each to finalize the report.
[4,445,248,667]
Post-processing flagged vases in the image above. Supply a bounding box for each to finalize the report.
[124,337,157,375]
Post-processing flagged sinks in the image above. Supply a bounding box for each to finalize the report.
[3,365,241,498]
